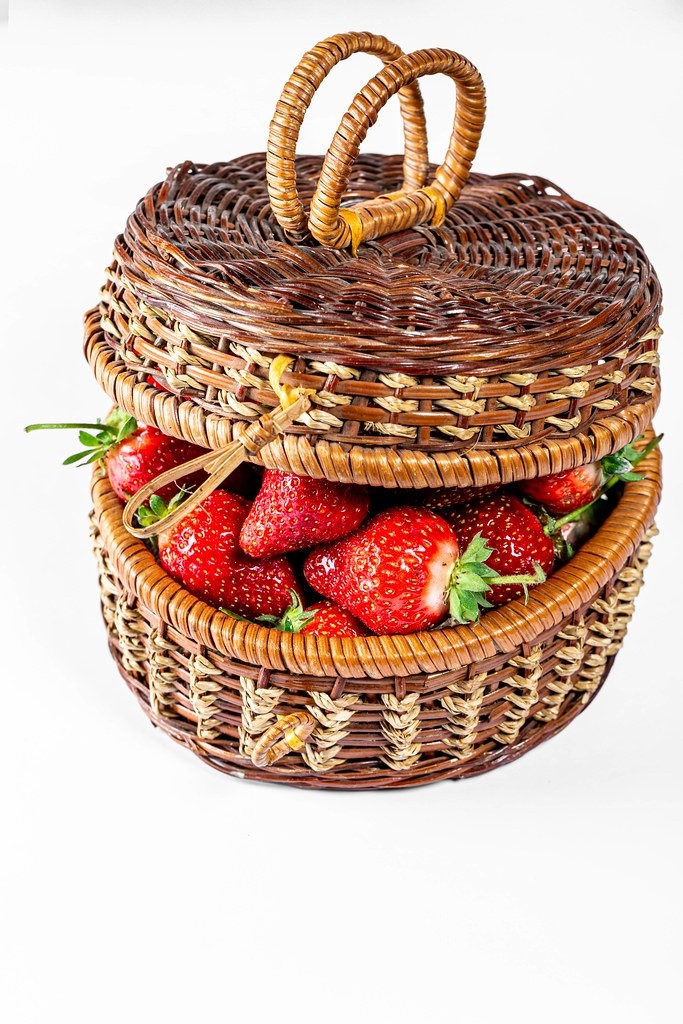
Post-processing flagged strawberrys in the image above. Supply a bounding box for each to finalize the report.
[26,416,664,639]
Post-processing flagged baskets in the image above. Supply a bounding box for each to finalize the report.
[79,31,660,796]
